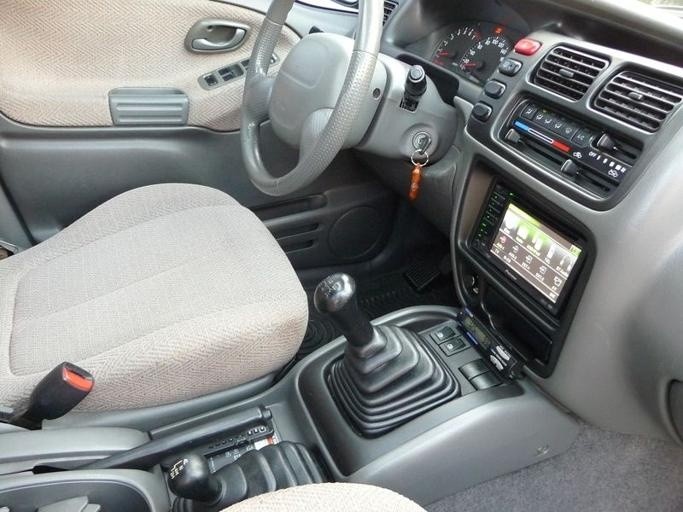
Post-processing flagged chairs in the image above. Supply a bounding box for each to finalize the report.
[1,465,429,512]
[1,182,310,467]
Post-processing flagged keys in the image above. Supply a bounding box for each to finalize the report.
[408,136,431,202]
